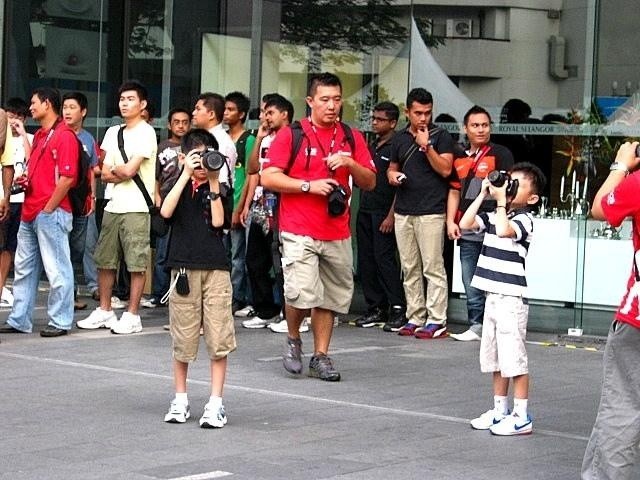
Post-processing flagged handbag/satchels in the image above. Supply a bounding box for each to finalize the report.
[151,207,169,237]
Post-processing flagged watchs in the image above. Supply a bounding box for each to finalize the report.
[608,161,629,177]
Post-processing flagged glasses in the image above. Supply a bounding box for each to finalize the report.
[372,116,389,123]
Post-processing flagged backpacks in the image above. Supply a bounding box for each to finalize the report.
[52,128,89,214]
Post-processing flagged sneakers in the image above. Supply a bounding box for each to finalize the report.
[283,337,303,373]
[309,357,340,381]
[235,305,339,332]
[356,307,481,341]
[0,289,156,336]
[470,410,532,436]
[164,400,190,423]
[199,404,227,428]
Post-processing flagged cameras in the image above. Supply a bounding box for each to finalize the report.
[10,176,29,195]
[190,145,225,171]
[398,175,410,184]
[328,184,348,216]
[486,169,519,196]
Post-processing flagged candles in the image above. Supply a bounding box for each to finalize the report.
[560,169,589,202]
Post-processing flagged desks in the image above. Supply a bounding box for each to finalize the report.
[452,208,638,314]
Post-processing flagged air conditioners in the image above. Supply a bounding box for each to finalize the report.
[445,18,474,40]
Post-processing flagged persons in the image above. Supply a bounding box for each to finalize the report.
[1,77,574,345]
[457,160,550,437]
[151,125,238,430]
[570,140,640,480]
[259,70,379,383]
